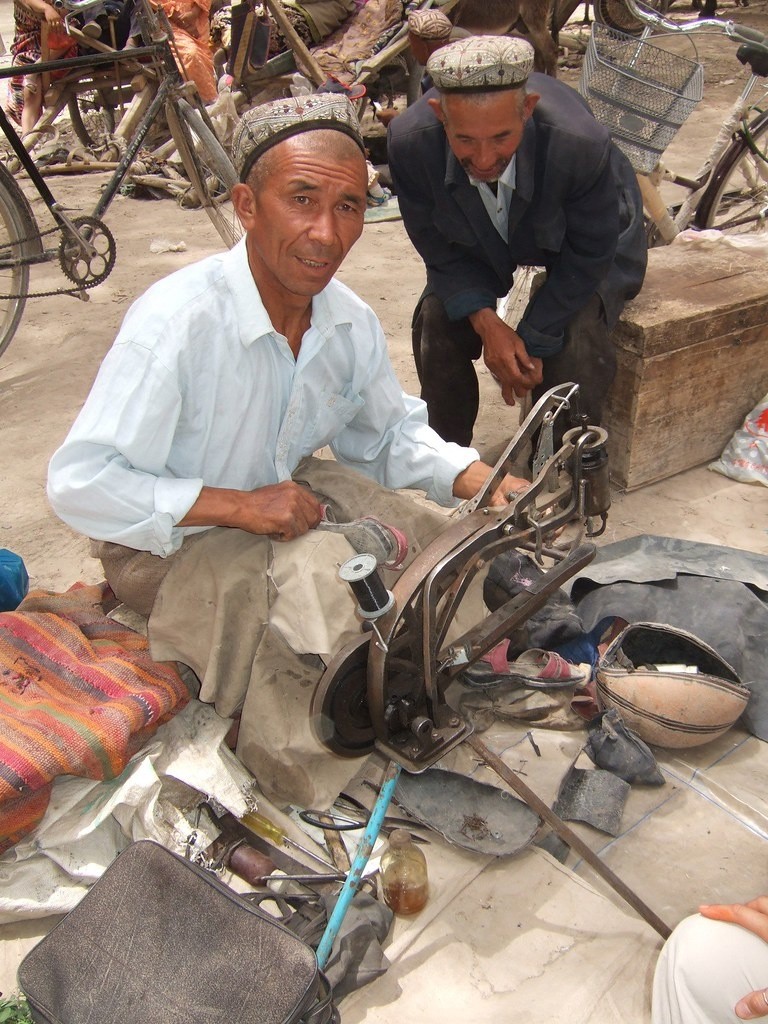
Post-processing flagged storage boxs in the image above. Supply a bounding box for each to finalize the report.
[526,238,767,493]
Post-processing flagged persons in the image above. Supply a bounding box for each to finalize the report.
[386,38,647,506]
[405,9,454,96]
[313,79,390,208]
[7,0,222,169]
[45,91,566,707]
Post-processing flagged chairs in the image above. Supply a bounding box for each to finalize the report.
[356,0,435,121]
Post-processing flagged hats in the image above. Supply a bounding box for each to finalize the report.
[231,92,366,185]
[427,35,536,92]
[408,8,452,40]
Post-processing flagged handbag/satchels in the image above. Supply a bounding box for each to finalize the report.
[227,0,273,87]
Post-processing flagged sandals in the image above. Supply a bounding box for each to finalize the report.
[316,503,407,572]
[457,639,585,688]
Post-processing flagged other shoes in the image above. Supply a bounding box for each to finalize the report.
[121,45,132,52]
[78,24,102,47]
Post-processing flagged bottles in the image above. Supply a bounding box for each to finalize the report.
[380,829,429,915]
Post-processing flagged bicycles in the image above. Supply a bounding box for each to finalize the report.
[574,0,768,252]
[0,0,247,363]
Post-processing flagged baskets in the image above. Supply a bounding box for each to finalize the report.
[578,22,704,174]
